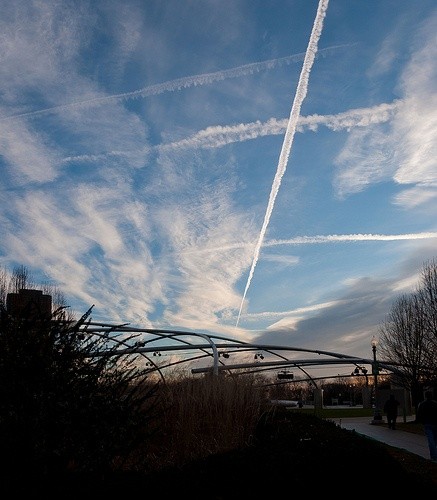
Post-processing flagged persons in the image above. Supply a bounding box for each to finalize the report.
[417,391,437,461]
[384,394,398,430]
